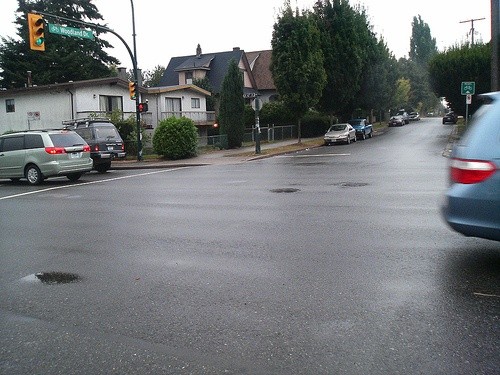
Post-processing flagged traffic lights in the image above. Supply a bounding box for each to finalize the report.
[27,13,45,51]
[139,103,148,112]
[129,82,137,100]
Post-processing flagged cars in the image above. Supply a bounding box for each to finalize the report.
[324,123,357,146]
[388,116,405,127]
[408,112,420,121]
[443,112,458,124]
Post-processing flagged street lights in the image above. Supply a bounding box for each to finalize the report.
[458,17,487,44]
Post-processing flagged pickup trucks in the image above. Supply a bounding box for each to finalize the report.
[346,119,373,140]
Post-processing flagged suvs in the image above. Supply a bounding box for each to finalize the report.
[0,128,93,185]
[439,90,500,242]
[395,109,410,124]
[62,117,127,172]
